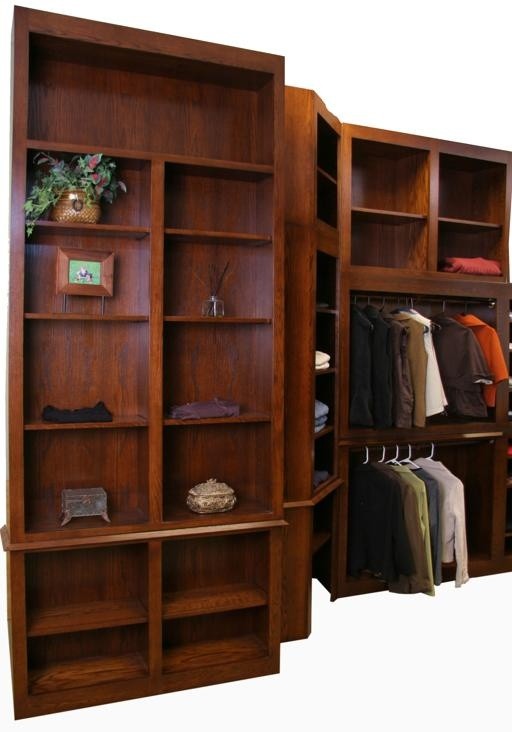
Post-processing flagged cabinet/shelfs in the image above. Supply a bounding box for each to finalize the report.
[1,7,288,720]
[283,83,512,640]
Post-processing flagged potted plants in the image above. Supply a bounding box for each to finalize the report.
[22,145,127,241]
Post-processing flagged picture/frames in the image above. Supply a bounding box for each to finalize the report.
[54,247,116,297]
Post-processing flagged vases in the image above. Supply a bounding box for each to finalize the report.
[200,296,225,318]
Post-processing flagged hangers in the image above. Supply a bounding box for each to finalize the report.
[360,443,437,472]
[350,292,474,336]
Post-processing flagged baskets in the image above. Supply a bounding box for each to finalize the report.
[50,189,102,223]
